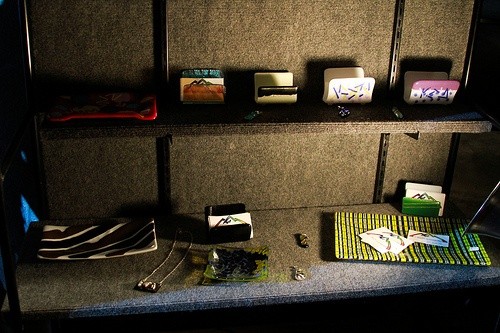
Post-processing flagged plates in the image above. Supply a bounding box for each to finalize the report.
[203,245,269,282]
[334,210,491,267]
[36,218,158,261]
[47,93,158,123]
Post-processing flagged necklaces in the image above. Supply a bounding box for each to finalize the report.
[132,226,194,293]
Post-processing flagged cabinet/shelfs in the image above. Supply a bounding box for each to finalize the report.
[0,0,500,332]
